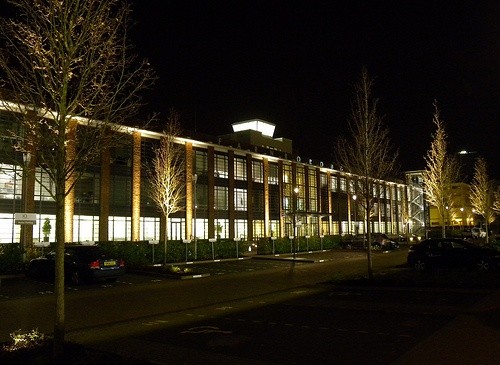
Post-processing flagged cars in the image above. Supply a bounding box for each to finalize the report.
[345,234,399,251]
[406,238,500,285]
[28,245,127,288]
